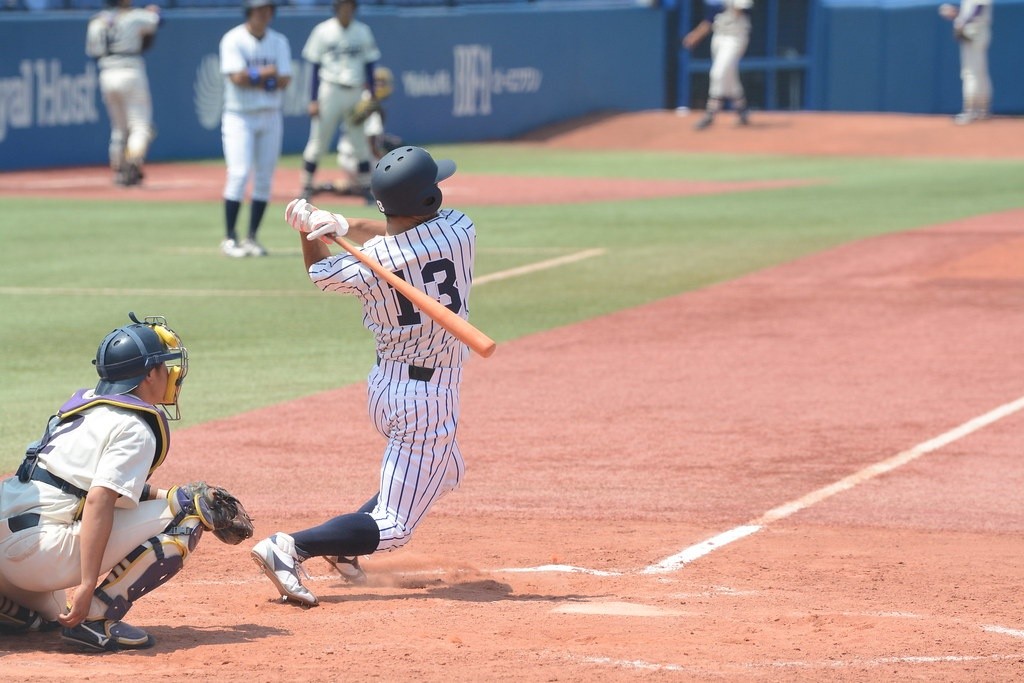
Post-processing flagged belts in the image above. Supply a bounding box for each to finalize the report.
[377,355,435,381]
[8,514,40,532]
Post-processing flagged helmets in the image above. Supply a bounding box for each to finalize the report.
[243,0,275,16]
[371,145,457,215]
[94,324,168,396]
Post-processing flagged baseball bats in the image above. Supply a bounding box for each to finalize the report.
[325,230,496,358]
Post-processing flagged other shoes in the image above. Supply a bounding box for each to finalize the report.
[223,238,247,257]
[695,117,711,131]
[739,108,751,126]
[957,110,988,124]
[362,187,374,201]
[245,239,265,256]
[299,188,313,203]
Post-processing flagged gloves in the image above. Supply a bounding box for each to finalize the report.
[285,198,313,233]
[307,210,349,245]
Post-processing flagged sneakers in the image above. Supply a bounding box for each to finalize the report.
[321,555,367,586]
[251,532,319,606]
[61,617,156,650]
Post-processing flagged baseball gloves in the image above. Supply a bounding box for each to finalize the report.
[210,487,254,547]
[353,99,387,125]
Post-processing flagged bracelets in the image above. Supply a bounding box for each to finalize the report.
[265,77,277,91]
[249,66,260,85]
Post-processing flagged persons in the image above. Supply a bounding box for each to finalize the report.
[338,66,400,180]
[0,312,255,649]
[299,0,381,203]
[219,0,293,257]
[683,0,754,130]
[939,0,992,122]
[252,146,477,611]
[85,0,161,186]
